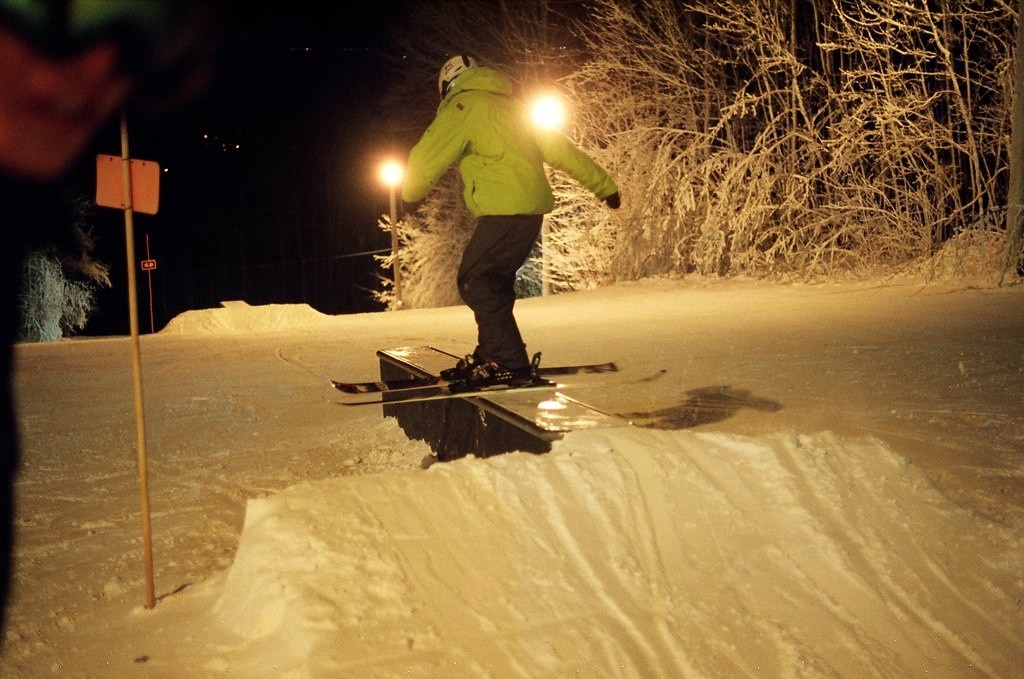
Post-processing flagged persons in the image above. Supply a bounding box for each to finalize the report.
[402,54,620,383]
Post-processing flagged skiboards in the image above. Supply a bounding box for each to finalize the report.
[328,356,668,407]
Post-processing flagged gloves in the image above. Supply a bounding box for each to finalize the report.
[402,199,425,216]
[600,192,621,209]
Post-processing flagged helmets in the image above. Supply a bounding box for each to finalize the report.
[438,55,477,101]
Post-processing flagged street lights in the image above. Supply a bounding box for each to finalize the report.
[381,159,405,308]
[534,99,563,297]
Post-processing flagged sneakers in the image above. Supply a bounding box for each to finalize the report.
[449,355,531,393]
[440,350,484,381]
